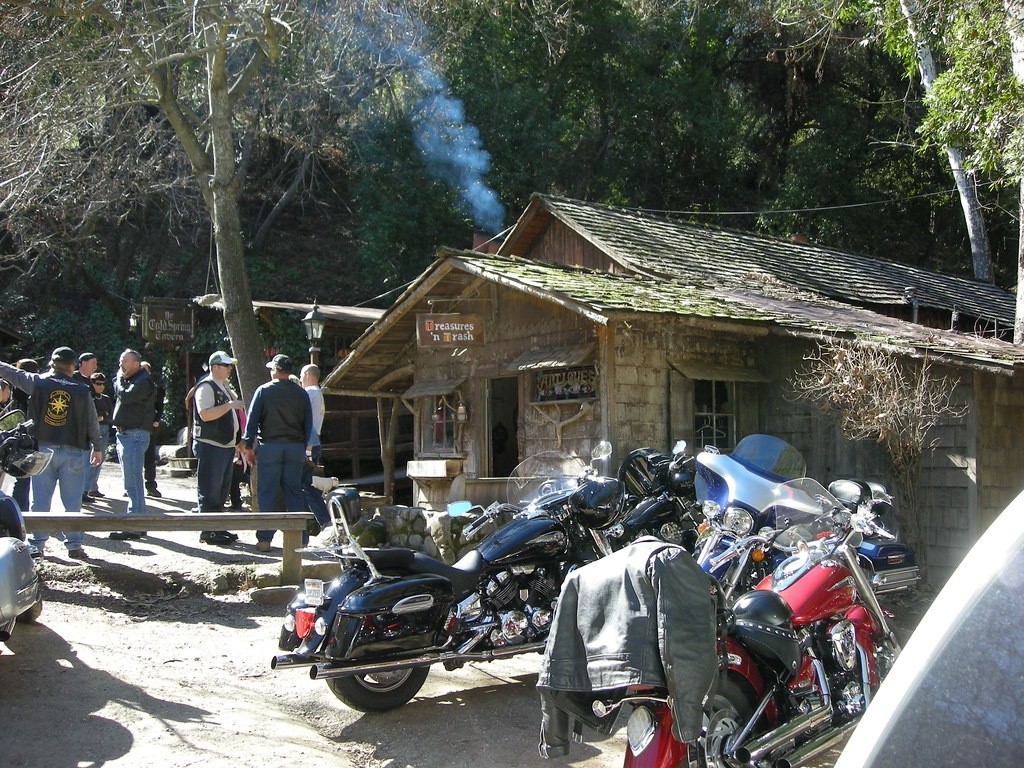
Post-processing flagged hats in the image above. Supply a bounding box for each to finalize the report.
[209,351,237,365]
[51,346,76,362]
[266,354,294,371]
[78,352,96,361]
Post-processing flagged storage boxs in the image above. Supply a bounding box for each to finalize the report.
[279,580,330,650]
[324,575,454,660]
[407,461,462,477]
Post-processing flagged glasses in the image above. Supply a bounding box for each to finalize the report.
[94,382,107,386]
[217,363,233,367]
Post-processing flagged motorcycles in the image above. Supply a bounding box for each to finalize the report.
[0,410,44,643]
[269,432,922,768]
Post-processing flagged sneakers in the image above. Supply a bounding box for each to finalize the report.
[302,543,308,547]
[256,541,270,552]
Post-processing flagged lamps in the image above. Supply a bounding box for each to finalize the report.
[129,313,143,332]
[457,403,468,421]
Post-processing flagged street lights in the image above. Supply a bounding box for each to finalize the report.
[303,298,326,364]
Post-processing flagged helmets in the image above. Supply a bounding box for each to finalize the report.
[732,589,801,676]
[567,477,625,530]
[0,424,40,479]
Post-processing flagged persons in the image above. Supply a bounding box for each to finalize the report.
[299,364,332,531]
[109,349,156,540]
[191,351,245,544]
[241,354,309,554]
[0,347,103,557]
[73,353,114,502]
[141,361,164,497]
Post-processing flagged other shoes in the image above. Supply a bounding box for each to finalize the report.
[140,531,148,537]
[146,489,162,497]
[224,501,241,511]
[109,531,140,540]
[191,507,199,513]
[123,493,127,497]
[30,550,43,557]
[88,491,105,496]
[82,494,96,502]
[68,547,84,558]
[199,531,238,545]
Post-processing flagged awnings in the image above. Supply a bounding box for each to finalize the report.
[401,377,467,398]
[506,342,596,371]
[668,359,771,382]
